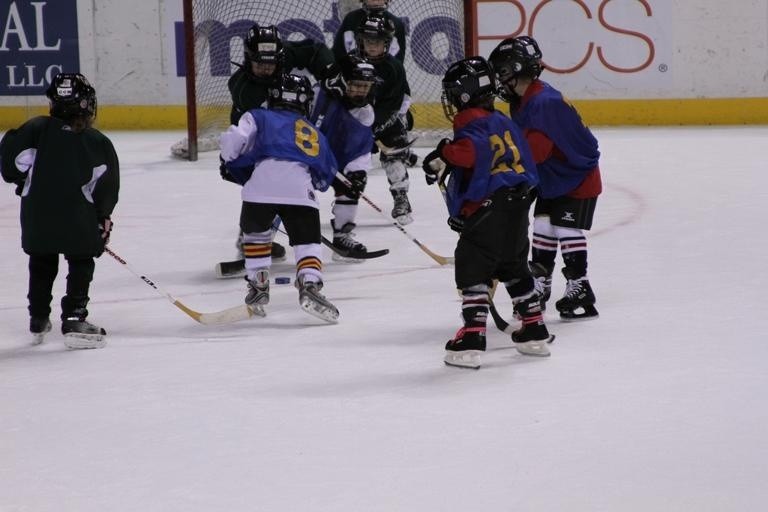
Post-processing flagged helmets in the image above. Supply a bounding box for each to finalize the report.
[352,14,395,54]
[439,55,496,123]
[488,35,546,104]
[330,55,382,106]
[45,72,98,125]
[243,23,286,69]
[266,71,315,114]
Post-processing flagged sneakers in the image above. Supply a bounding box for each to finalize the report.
[244,269,270,306]
[59,316,108,338]
[238,239,286,258]
[329,218,369,253]
[555,263,596,312]
[527,261,552,300]
[389,189,412,218]
[28,311,51,334]
[511,293,550,344]
[293,273,340,318]
[444,305,489,353]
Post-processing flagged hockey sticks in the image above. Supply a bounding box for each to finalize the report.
[430,160,556,344]
[320,233,390,260]
[375,136,419,155]
[335,170,454,267]
[214,95,332,279]
[104,246,255,328]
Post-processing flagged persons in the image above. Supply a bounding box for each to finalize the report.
[0,73,121,347]
[219,75,339,323]
[228,25,290,259]
[488,36,602,318]
[326,11,416,222]
[422,55,551,370]
[332,1,406,61]
[309,50,375,262]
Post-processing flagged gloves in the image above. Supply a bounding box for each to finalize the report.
[422,136,452,187]
[340,170,369,201]
